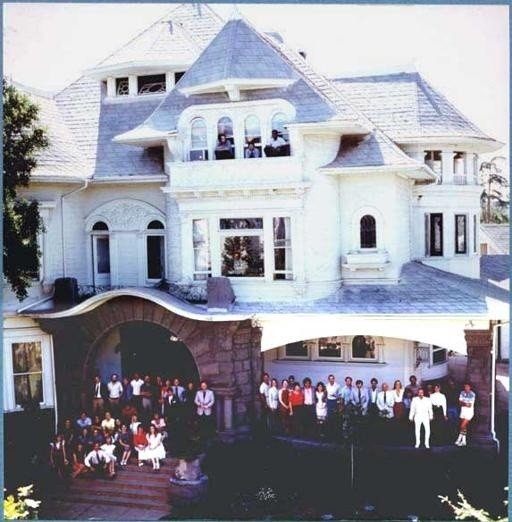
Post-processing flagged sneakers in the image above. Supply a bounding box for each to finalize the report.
[108,472,116,478]
[454,440,467,447]
[152,462,160,471]
[120,461,126,465]
[425,443,430,449]
[415,444,420,449]
[138,462,144,467]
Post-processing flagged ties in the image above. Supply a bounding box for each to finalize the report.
[96,386,98,397]
[96,453,101,463]
[359,388,361,404]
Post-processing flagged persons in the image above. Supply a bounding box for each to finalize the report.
[453,384,476,447]
[232,252,248,275]
[448,349,455,357]
[266,129,286,149]
[244,142,259,157]
[257,373,449,451]
[214,133,233,154]
[47,371,216,481]
